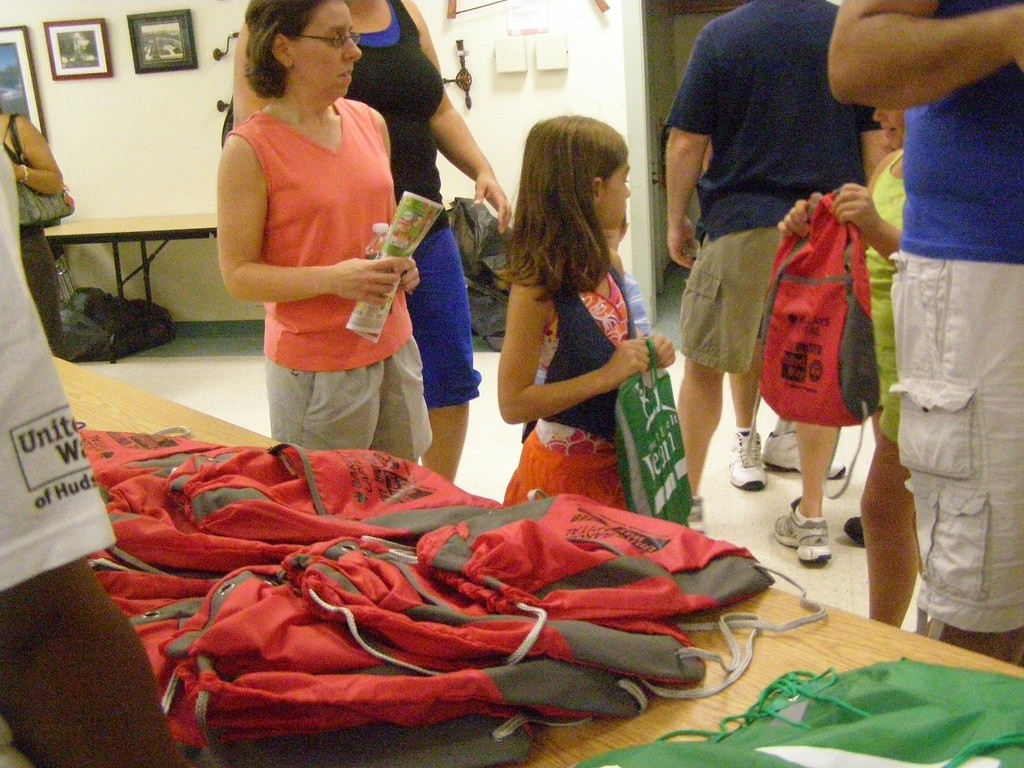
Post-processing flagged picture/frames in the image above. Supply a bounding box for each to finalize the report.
[0,25,49,144]
[43,18,113,81]
[127,9,198,74]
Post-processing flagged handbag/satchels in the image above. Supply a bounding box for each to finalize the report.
[614,340,692,527]
[7,114,75,226]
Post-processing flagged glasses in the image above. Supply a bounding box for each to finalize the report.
[294,34,361,49]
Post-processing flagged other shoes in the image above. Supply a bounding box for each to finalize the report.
[843,516,863,546]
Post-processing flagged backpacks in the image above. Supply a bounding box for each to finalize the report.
[82,426,1024,768]
[758,192,881,501]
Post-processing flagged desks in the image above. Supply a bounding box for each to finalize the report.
[42,213,218,364]
[51,354,1024,768]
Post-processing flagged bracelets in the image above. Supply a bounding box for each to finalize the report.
[16,163,28,184]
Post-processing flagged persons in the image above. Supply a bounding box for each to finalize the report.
[829,1,1024,666]
[0,102,67,360]
[218,0,516,486]
[661,0,922,628]
[0,186,192,768]
[500,126,676,510]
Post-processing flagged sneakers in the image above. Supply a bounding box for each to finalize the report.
[763,421,846,480]
[728,430,767,489]
[771,496,831,562]
